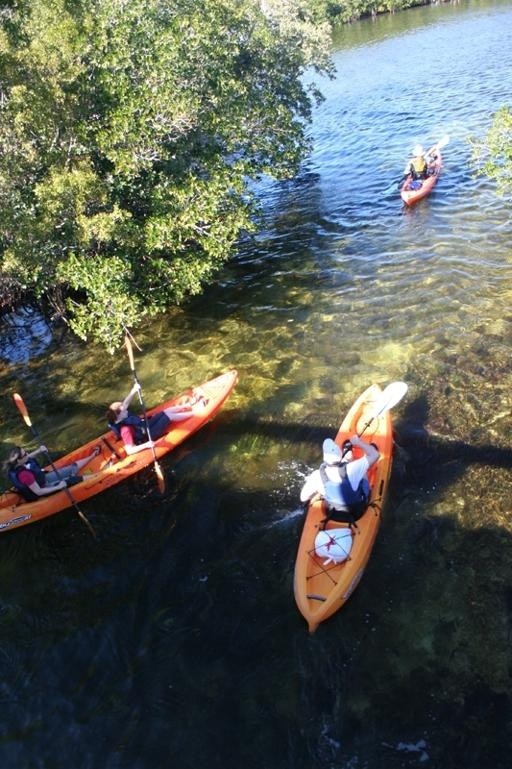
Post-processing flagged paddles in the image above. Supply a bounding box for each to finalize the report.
[124,335,177,498]
[11,389,104,547]
[341,378,407,459]
[426,133,453,156]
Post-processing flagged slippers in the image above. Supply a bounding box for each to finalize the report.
[94,446,100,455]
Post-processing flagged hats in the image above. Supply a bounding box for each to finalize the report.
[323,438,342,464]
[412,146,424,156]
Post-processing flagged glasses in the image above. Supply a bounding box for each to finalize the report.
[21,452,27,458]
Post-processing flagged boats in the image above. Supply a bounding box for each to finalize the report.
[291,379,399,636]
[0,370,238,536]
[398,147,444,205]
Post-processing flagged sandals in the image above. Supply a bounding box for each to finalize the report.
[343,440,352,458]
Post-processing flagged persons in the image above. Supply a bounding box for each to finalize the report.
[4,445,115,504]
[106,383,210,456]
[403,144,439,181]
[300,434,381,524]
